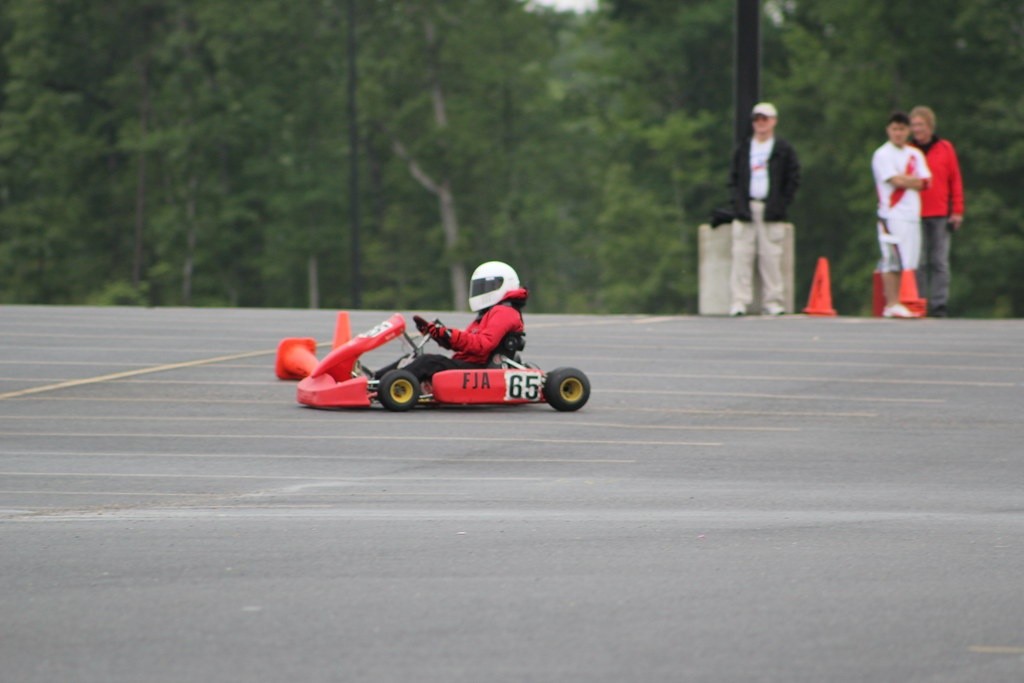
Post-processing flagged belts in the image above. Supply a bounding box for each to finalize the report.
[748,196,768,203]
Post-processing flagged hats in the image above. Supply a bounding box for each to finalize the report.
[752,102,778,117]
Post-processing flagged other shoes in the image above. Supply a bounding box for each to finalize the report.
[730,303,747,316]
[355,360,373,381]
[761,302,785,316]
[933,304,948,317]
[883,303,921,318]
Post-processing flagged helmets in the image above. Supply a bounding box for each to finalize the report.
[468,260,520,312]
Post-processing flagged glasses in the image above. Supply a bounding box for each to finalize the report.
[754,116,776,121]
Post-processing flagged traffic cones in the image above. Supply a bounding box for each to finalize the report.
[898,269,927,318]
[802,257,835,316]
[275,337,320,381]
[330,310,352,351]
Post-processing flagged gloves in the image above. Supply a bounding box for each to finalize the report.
[418,321,434,335]
[427,321,452,350]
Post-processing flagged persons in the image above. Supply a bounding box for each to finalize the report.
[871,114,934,319]
[353,260,528,384]
[729,101,801,316]
[906,105,965,318]
